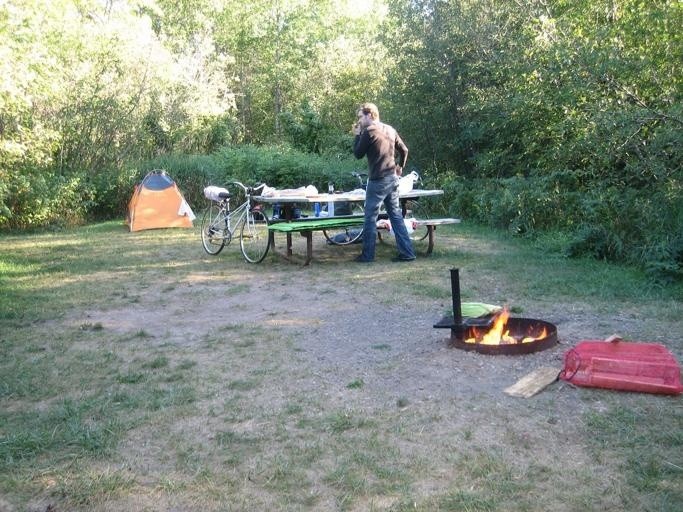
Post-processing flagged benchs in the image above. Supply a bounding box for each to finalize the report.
[248,214,462,268]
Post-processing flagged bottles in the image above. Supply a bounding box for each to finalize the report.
[328,181,333,194]
[314,203,320,218]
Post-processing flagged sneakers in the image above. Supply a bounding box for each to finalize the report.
[391,253,416,262]
[353,254,365,262]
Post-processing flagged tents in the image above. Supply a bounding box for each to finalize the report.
[123,168,195,232]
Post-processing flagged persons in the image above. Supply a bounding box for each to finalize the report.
[351,102,417,263]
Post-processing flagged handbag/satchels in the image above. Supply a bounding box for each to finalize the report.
[333,202,353,215]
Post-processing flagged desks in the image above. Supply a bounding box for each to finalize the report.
[248,190,444,271]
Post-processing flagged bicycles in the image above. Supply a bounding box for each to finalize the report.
[200,178,272,264]
[320,170,431,247]
